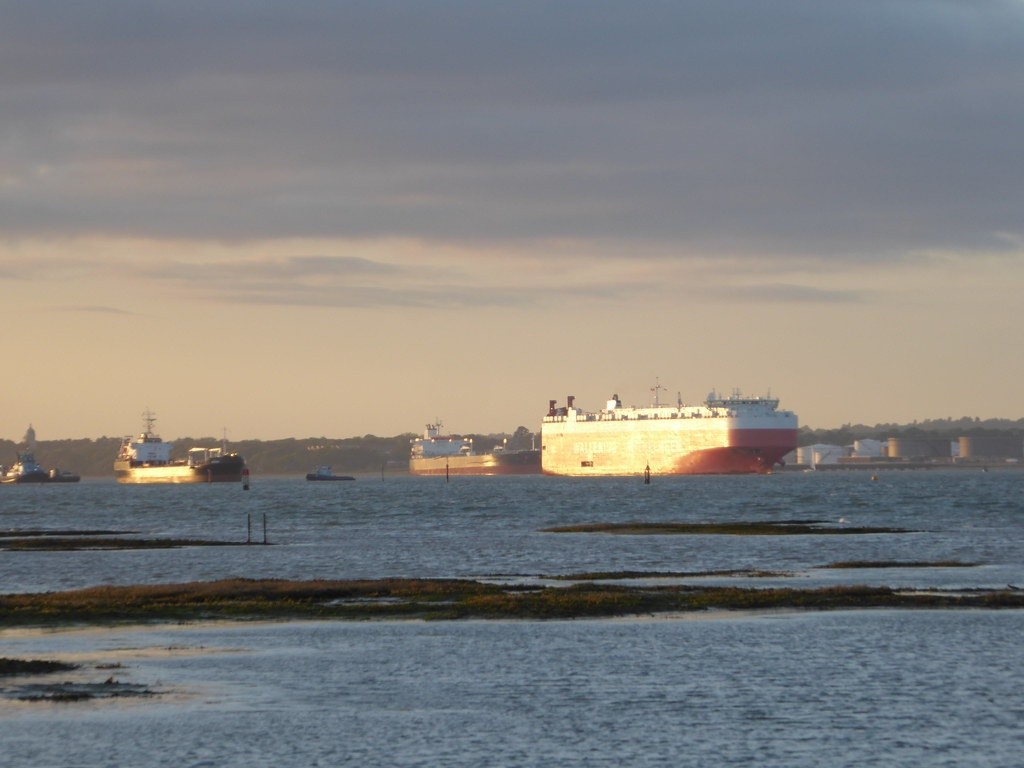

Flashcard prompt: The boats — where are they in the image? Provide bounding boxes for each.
[409,424,522,475]
[0,445,81,484]
[305,465,356,481]
[541,395,798,476]
[112,409,246,485]
